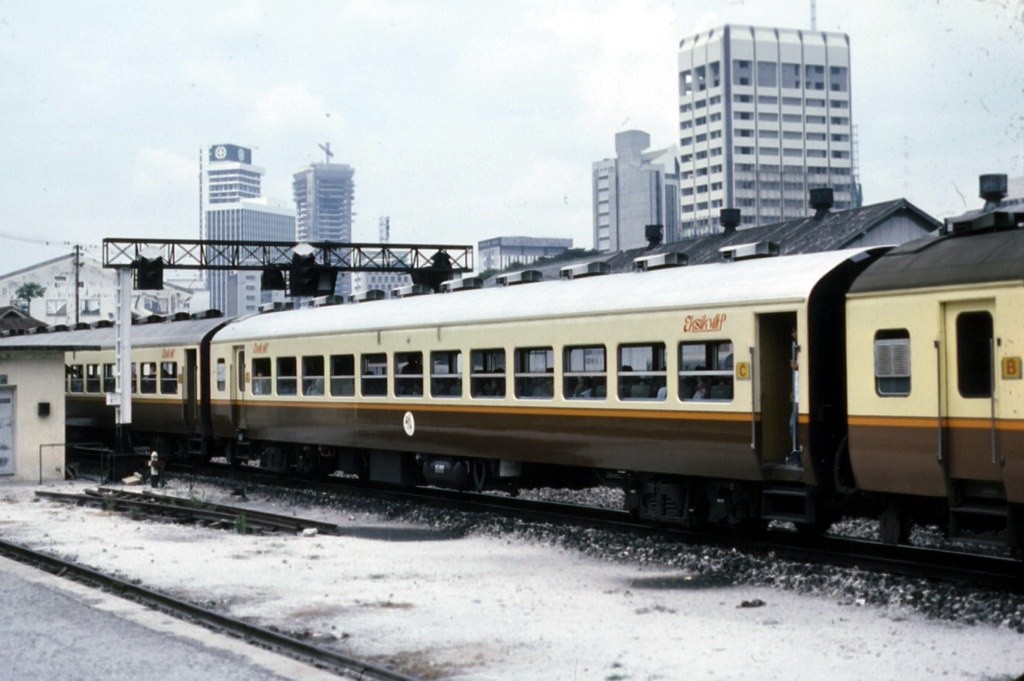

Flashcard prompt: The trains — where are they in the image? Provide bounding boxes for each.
[65,209,1024,544]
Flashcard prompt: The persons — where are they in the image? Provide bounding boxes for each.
[70,356,713,405]
